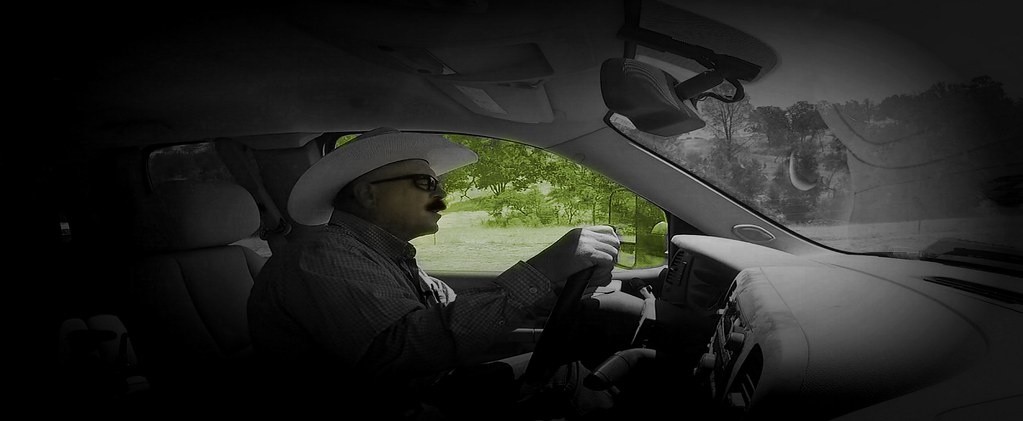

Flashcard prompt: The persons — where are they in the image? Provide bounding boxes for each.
[277,126,620,421]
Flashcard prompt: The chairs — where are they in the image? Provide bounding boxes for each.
[144,176,274,355]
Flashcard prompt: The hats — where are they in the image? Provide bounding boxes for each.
[287,126,478,226]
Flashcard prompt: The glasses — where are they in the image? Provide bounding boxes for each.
[365,173,441,192]
